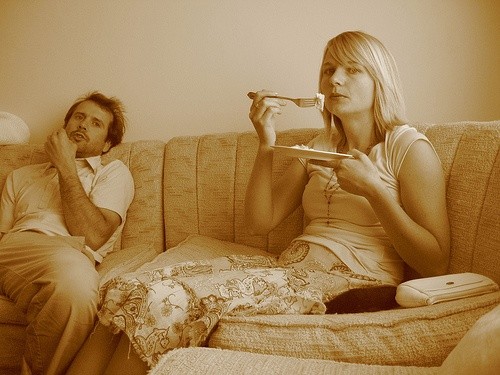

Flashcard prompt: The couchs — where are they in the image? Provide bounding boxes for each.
[0,121,500,375]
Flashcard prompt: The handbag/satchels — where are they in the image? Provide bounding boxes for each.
[395,272,498,308]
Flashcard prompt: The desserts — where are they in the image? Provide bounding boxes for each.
[315,92,325,112]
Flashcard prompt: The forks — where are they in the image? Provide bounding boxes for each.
[247,92,317,108]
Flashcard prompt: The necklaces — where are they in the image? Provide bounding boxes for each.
[323,143,374,223]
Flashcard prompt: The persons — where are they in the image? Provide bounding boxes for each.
[0,93,135,375]
[66,31,452,375]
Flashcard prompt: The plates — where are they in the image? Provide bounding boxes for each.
[270,146,355,161]
